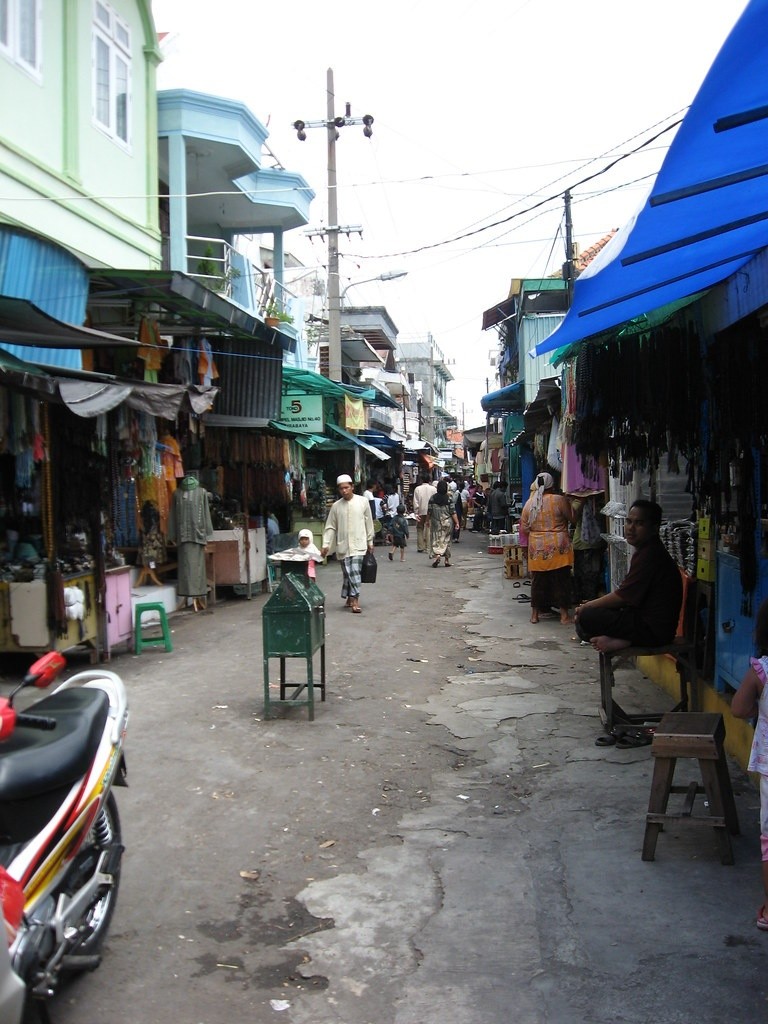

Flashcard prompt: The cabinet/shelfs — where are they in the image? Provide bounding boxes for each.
[0,565,134,665]
[713,551,768,728]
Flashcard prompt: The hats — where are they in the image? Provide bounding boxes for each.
[180,476,199,491]
[337,474,352,485]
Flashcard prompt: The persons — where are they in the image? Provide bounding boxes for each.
[425,480,460,568]
[322,474,375,613]
[519,472,602,625]
[362,478,400,545]
[414,471,514,553]
[298,529,330,585]
[574,500,684,654]
[731,597,768,931]
[387,505,410,563]
[168,477,213,598]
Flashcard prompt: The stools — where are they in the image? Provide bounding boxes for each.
[133,602,171,654]
[641,712,741,867]
[597,637,699,736]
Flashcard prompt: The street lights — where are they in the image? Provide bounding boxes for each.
[327,271,410,383]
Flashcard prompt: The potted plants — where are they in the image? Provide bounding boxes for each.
[261,293,280,327]
[277,309,305,338]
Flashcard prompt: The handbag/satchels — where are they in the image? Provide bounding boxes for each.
[361,552,378,583]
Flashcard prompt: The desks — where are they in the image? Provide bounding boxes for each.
[693,580,715,681]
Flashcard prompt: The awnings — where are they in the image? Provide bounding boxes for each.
[282,366,401,409]
[462,424,503,449]
[483,296,515,329]
[527,0,768,356]
[509,375,563,447]
[199,411,409,464]
[0,267,299,421]
[481,379,525,411]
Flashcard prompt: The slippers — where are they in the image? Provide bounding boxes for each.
[512,593,532,603]
[523,580,531,586]
[595,727,652,748]
[756,904,768,929]
[538,613,560,618]
[513,582,520,588]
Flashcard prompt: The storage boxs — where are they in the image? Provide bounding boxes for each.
[697,517,716,582]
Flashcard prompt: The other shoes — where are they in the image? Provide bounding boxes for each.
[346,600,353,607]
[352,605,361,612]
[432,556,441,568]
[445,562,451,567]
[388,552,393,561]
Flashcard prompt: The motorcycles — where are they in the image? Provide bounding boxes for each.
[0,640,129,1024]
